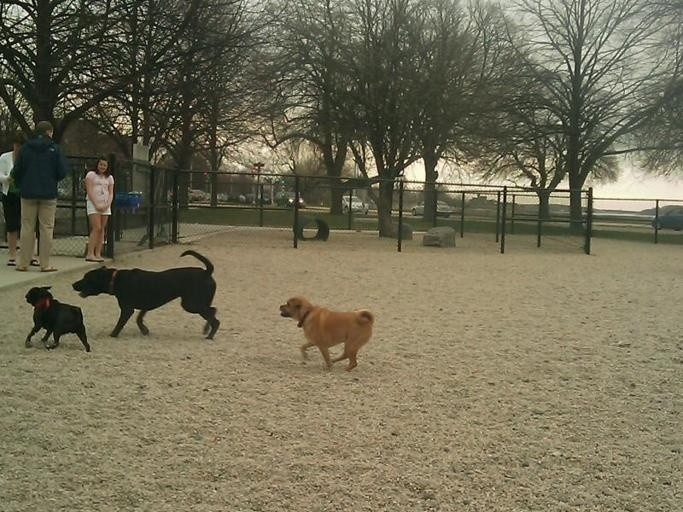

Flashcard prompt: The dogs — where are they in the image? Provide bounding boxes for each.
[72,250,220,339]
[280,297,374,372]
[26,287,89,352]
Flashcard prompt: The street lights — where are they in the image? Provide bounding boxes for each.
[254,162,265,203]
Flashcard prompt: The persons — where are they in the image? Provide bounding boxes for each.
[84,155,116,263]
[7,119,69,272]
[0,130,41,266]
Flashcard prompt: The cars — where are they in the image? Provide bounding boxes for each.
[343,196,368,213]
[188,188,303,208]
[652,208,683,230]
[410,200,455,218]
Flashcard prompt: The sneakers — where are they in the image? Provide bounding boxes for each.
[16,266,27,271]
[31,259,40,265]
[8,260,16,265]
[85,256,104,262]
[41,266,57,272]
